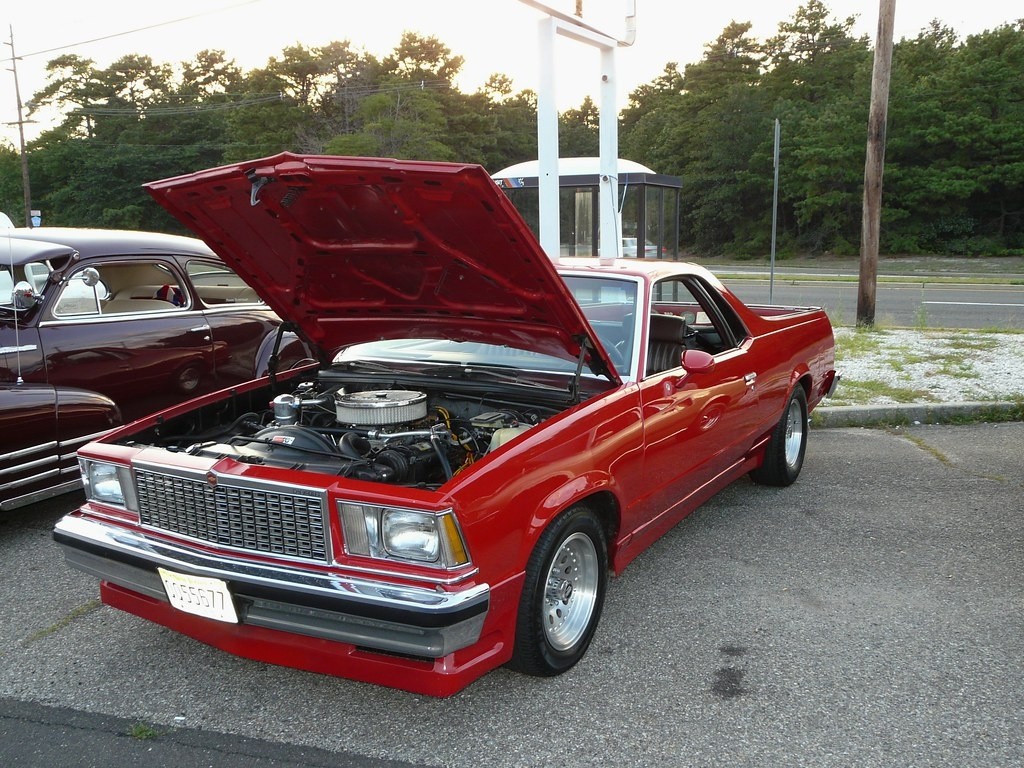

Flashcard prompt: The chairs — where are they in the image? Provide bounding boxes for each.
[622,311,687,374]
[56,297,108,313]
[101,300,179,312]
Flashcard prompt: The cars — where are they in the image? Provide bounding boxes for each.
[0,212,106,303]
[52,151,842,700]
[597,238,667,260]
[0,227,319,512]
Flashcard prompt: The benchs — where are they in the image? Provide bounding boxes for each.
[115,284,259,304]
